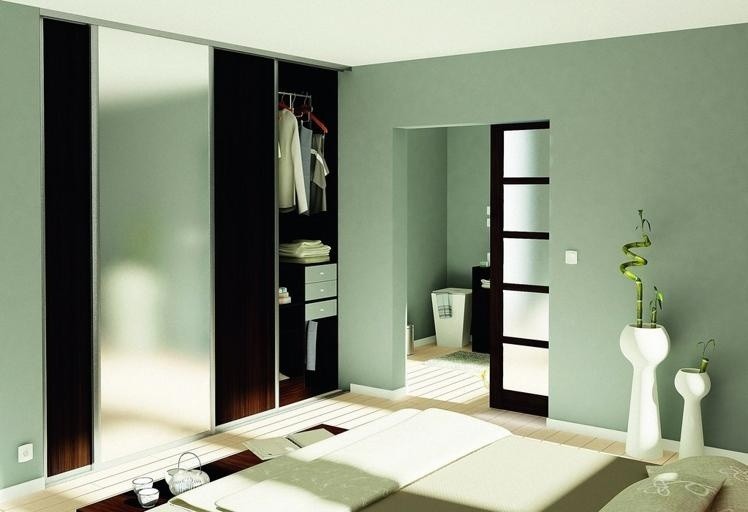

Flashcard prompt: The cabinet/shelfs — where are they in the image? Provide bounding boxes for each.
[0,0,355,496]
[471,265,490,356]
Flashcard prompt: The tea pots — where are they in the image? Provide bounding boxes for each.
[168,451,210,495]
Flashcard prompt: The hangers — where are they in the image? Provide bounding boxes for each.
[278,90,329,137]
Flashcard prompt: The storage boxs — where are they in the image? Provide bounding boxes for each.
[429,286,472,349]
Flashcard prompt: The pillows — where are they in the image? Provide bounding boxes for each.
[644,454,747,512]
[597,471,728,511]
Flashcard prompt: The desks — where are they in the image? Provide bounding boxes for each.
[73,423,351,512]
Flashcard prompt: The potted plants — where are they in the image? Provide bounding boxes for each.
[617,208,673,461]
[674,338,718,461]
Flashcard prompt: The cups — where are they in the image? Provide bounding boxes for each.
[132,467,186,508]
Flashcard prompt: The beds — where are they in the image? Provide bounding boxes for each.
[143,407,748,512]
